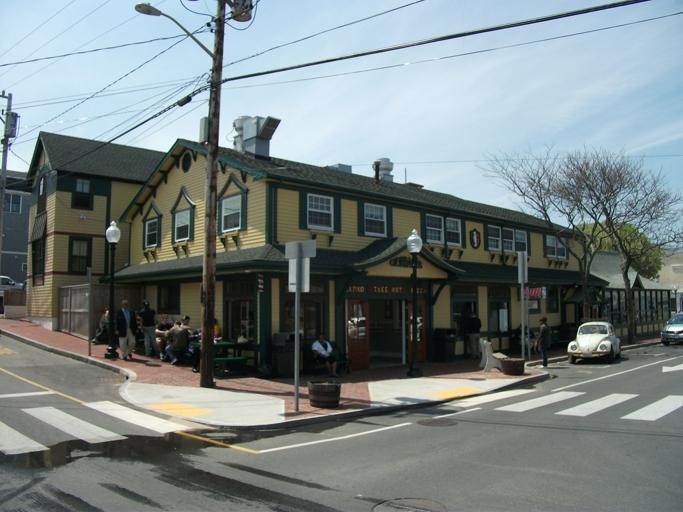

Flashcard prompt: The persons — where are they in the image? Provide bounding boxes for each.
[312,333,342,379]
[534,317,548,369]
[465,310,482,363]
[90,305,110,344]
[164,320,185,366]
[213,318,220,343]
[512,322,540,357]
[138,299,160,357]
[115,299,140,361]
[154,312,173,360]
[179,314,193,334]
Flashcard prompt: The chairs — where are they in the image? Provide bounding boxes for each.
[478,338,511,373]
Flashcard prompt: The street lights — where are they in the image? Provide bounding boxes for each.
[135,3,224,387]
[407,228,423,377]
[105,220,121,361]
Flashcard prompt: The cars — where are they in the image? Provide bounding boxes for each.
[0,276,24,296]
[661,311,683,346]
[567,321,621,364]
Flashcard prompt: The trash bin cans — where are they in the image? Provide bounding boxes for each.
[434,328,467,362]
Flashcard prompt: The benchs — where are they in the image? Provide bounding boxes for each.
[214,356,249,378]
[304,341,354,376]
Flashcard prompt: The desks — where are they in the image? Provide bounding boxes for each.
[189,339,236,377]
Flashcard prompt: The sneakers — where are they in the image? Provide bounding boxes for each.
[170,357,179,365]
[160,353,165,360]
[192,367,199,373]
[124,353,133,360]
